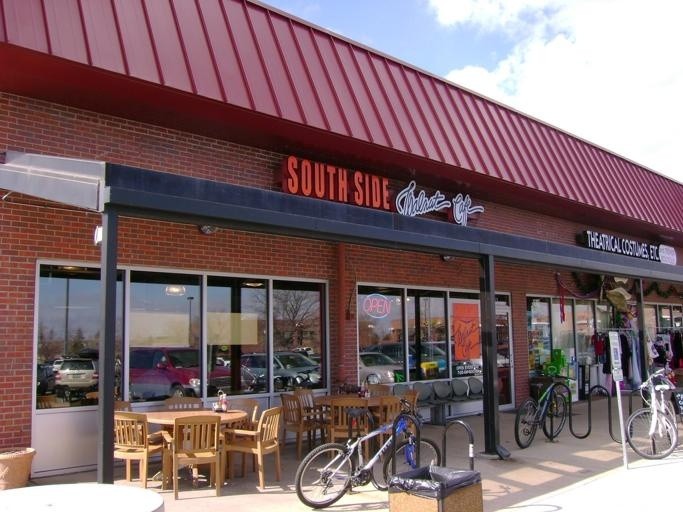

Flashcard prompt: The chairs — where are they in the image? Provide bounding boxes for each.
[393,377,484,426]
[113,397,285,500]
[280,383,420,469]
[36,391,99,409]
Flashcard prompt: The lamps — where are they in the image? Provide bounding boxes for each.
[164,285,186,297]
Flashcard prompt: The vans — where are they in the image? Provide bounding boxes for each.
[227,352,321,387]
[532,315,679,331]
[122,348,243,398]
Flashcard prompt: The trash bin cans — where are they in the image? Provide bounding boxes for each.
[388,466,483,512]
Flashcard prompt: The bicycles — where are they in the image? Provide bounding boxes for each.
[514,373,576,449]
[295,398,440,508]
[625,367,679,460]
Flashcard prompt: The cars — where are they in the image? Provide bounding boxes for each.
[54,358,98,396]
[298,347,313,352]
[359,339,508,385]
[53,360,63,372]
[37,367,55,394]
